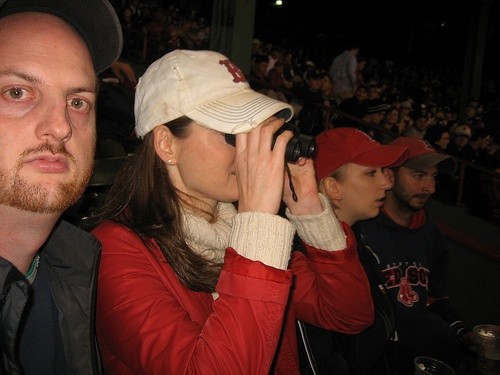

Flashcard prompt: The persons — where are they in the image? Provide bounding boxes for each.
[0,11,103,375]
[88,50,374,375]
[351,136,500,375]
[83,36,500,238]
[296,129,412,375]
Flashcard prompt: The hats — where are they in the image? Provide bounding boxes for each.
[417,103,427,110]
[400,102,413,111]
[133,49,294,139]
[454,125,471,138]
[388,137,457,172]
[306,69,324,81]
[312,127,408,181]
[0,0,124,73]
[365,98,386,113]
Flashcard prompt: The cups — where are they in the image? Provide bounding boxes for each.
[414,356,454,375]
[473,324,500,375]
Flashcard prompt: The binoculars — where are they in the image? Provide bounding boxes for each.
[224,119,316,162]
